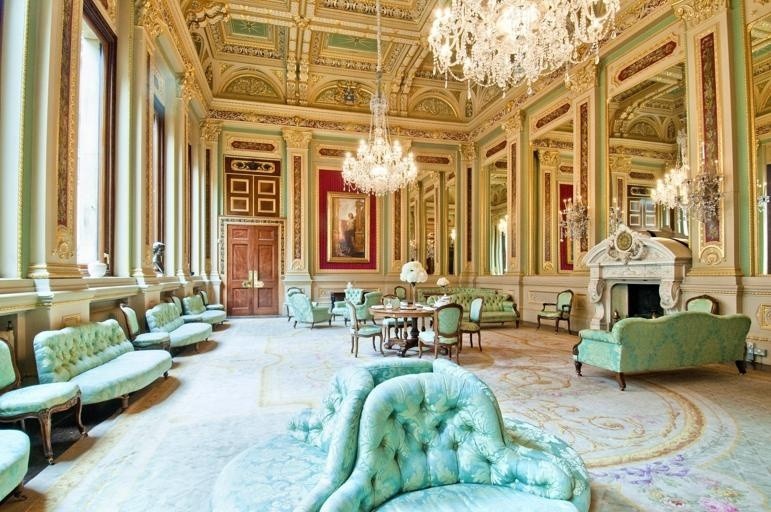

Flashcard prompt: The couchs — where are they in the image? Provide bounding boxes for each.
[183,294,226,331]
[0,430,31,502]
[33,318,172,409]
[144,302,212,353]
[572,314,752,391]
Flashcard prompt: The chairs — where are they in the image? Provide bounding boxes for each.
[200,291,223,325]
[167,295,202,323]
[120,303,171,352]
[685,295,720,315]
[0,337,85,465]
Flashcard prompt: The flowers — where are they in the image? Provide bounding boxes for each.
[401,262,428,283]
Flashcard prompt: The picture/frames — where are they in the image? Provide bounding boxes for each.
[327,192,370,263]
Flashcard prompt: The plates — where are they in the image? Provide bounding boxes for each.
[371,301,430,310]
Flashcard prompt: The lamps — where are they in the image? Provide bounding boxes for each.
[688,164,725,218]
[757,179,770,213]
[428,0,621,99]
[341,0,417,196]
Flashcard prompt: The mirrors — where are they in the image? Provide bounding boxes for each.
[740,0,771,276]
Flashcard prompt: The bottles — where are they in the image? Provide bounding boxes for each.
[88,260,109,278]
[346,281,354,288]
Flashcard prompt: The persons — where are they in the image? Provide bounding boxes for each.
[151,241,165,273]
[339,212,360,259]
